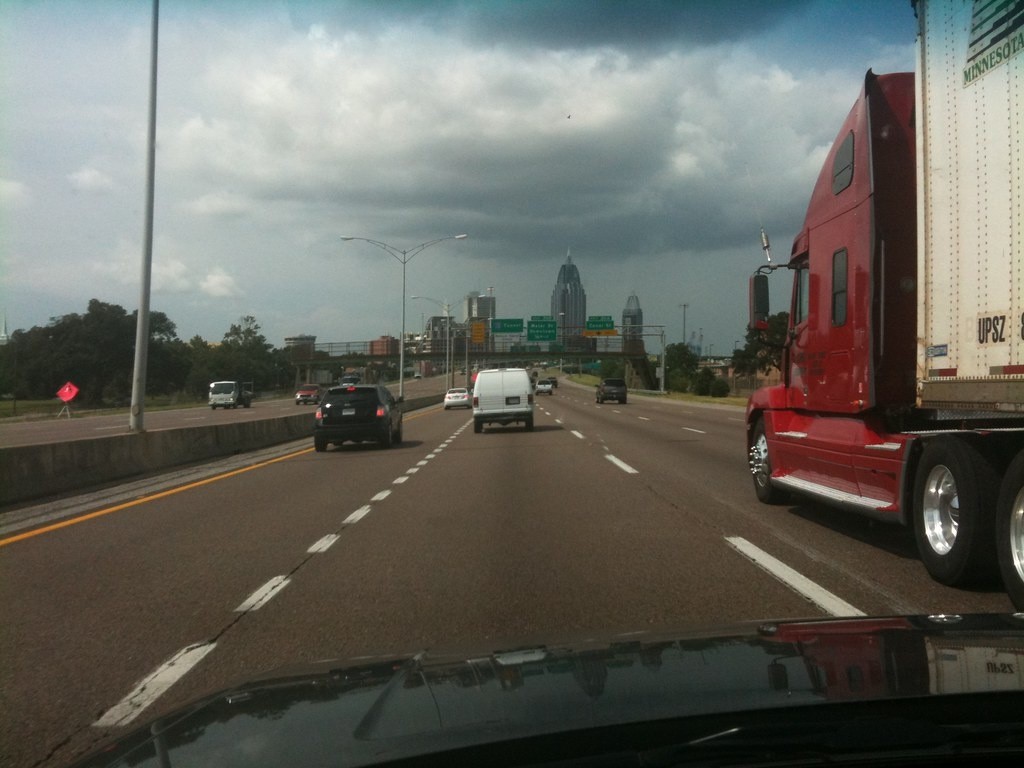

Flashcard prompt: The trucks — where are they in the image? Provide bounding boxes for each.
[208,380,254,409]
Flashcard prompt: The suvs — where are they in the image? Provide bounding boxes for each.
[594,378,628,405]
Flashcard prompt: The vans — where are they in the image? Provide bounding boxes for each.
[338,374,362,389]
[467,367,537,433]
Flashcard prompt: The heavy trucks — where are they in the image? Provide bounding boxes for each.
[744,0,1024,627]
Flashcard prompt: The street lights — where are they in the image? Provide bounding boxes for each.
[340,233,469,403]
[410,294,490,393]
[558,312,566,373]
[440,316,493,389]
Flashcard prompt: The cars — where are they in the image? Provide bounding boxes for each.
[531,359,559,371]
[547,376,559,388]
[529,370,538,387]
[294,383,326,406]
[443,387,473,410]
[534,378,553,396]
[414,372,423,380]
[314,383,406,450]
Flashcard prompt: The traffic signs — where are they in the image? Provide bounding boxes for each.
[527,319,557,341]
